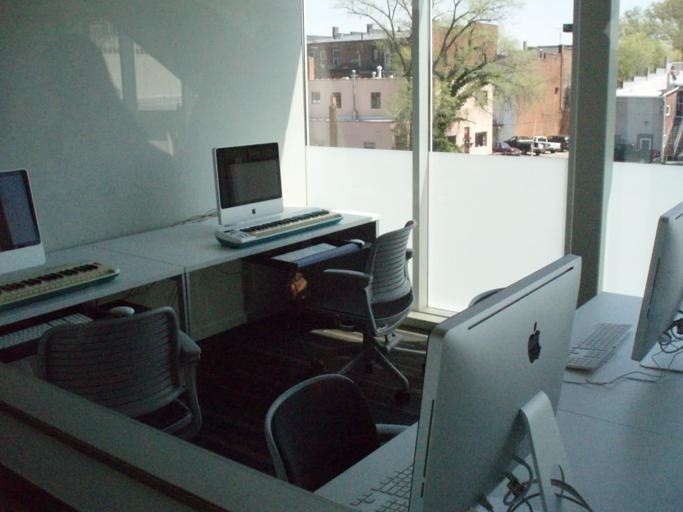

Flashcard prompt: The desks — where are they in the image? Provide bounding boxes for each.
[310,293,682,512]
[0,243,188,372]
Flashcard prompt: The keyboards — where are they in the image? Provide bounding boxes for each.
[565,321,633,374]
[0,311,94,351]
[270,242,337,264]
[341,456,415,512]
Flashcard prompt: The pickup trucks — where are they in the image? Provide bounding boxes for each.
[491,132,569,156]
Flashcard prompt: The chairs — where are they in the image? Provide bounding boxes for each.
[299,219,419,400]
[91,206,379,340]
[267,373,412,492]
[37,305,203,440]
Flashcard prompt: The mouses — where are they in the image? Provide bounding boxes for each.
[345,238,365,247]
[110,306,136,317]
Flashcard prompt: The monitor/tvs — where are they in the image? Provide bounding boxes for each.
[408,252,597,512]
[0,168,47,281]
[213,141,283,226]
[630,202,683,373]
